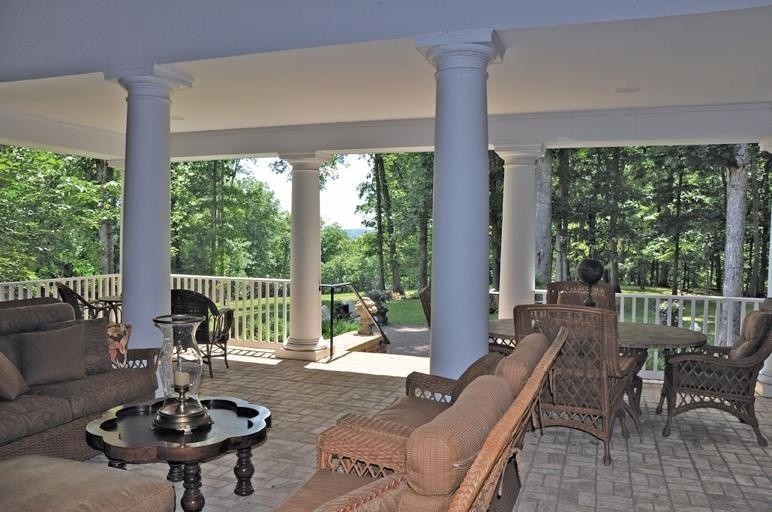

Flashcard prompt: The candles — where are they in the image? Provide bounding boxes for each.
[173,369,189,387]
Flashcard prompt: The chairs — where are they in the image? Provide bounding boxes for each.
[546,280,648,410]
[59,279,125,324]
[272,373,543,511]
[511,302,646,465]
[651,292,772,452]
[335,319,569,510]
[167,287,235,381]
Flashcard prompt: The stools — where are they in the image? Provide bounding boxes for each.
[0,451,175,510]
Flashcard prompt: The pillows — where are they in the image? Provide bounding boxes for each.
[1,316,134,399]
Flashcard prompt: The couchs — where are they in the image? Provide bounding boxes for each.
[2,296,163,462]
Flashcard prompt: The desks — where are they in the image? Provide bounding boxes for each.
[99,294,124,306]
[479,321,712,437]
